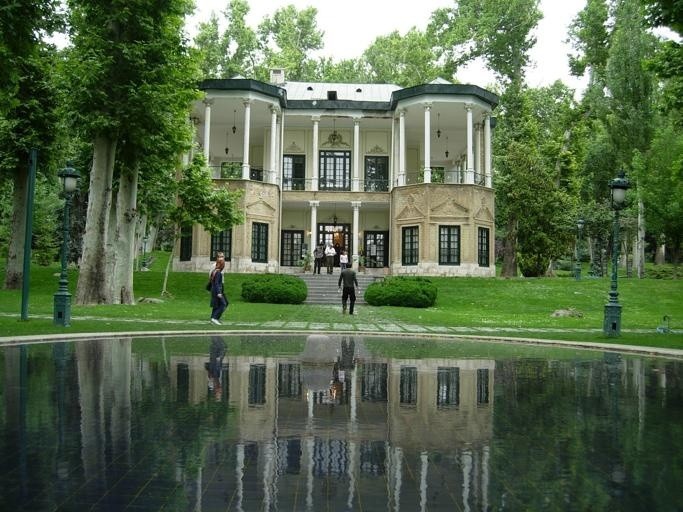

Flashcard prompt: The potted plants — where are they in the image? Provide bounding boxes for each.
[354,254,368,274]
[297,249,311,272]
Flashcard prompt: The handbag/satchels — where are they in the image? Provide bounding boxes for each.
[206,280,212,291]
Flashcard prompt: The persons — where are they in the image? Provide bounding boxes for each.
[338,262,361,316]
[207,251,229,327]
[327,336,356,407]
[203,334,228,405]
[313,237,348,276]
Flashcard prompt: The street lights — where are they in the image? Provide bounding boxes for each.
[53,160,84,328]
[603,168,632,341]
[139,236,148,273]
[575,219,584,279]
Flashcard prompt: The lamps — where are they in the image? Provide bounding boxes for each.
[436,112,442,139]
[445,136,449,158]
[232,109,237,134]
[224,132,229,155]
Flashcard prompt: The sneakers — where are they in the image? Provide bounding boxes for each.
[208,317,222,326]
